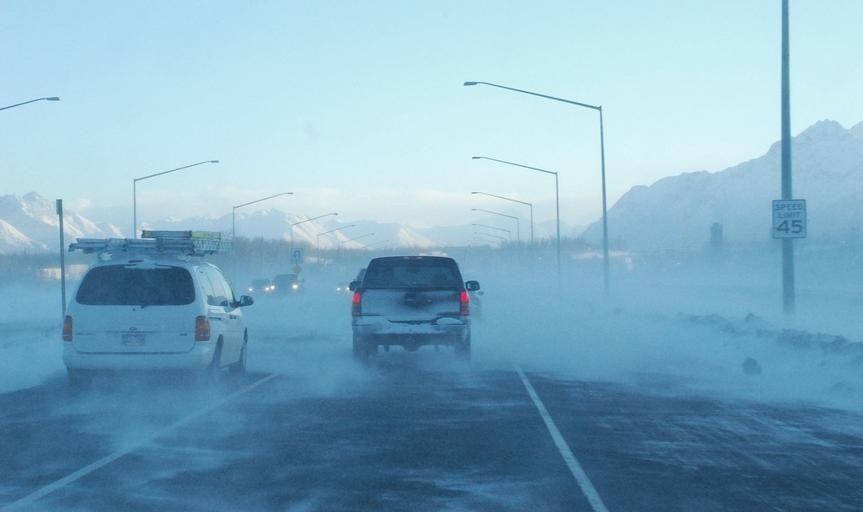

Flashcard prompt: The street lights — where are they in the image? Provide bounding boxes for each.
[232,191,294,237]
[341,232,375,252]
[316,224,356,263]
[463,81,612,306]
[0,97,60,111]
[133,159,220,236]
[290,213,338,246]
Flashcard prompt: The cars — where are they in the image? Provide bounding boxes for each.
[245,273,302,297]
[335,279,350,293]
[61,230,255,390]
[349,255,485,363]
